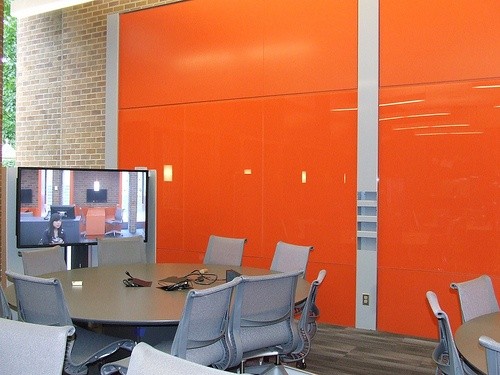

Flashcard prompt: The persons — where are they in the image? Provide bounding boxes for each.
[40,213,67,244]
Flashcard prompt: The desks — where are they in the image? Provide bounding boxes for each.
[454,311,500,375]
[86,208,105,239]
[6,262,314,345]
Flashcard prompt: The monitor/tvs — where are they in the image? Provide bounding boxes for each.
[50,205,76,219]
[16,166,149,244]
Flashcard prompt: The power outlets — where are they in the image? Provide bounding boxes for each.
[363,294,369,305]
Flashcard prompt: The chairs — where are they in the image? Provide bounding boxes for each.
[450,276,499,325]
[105,207,125,238]
[425,290,477,375]
[0,234,327,375]
[478,336,500,375]
[78,205,86,238]
